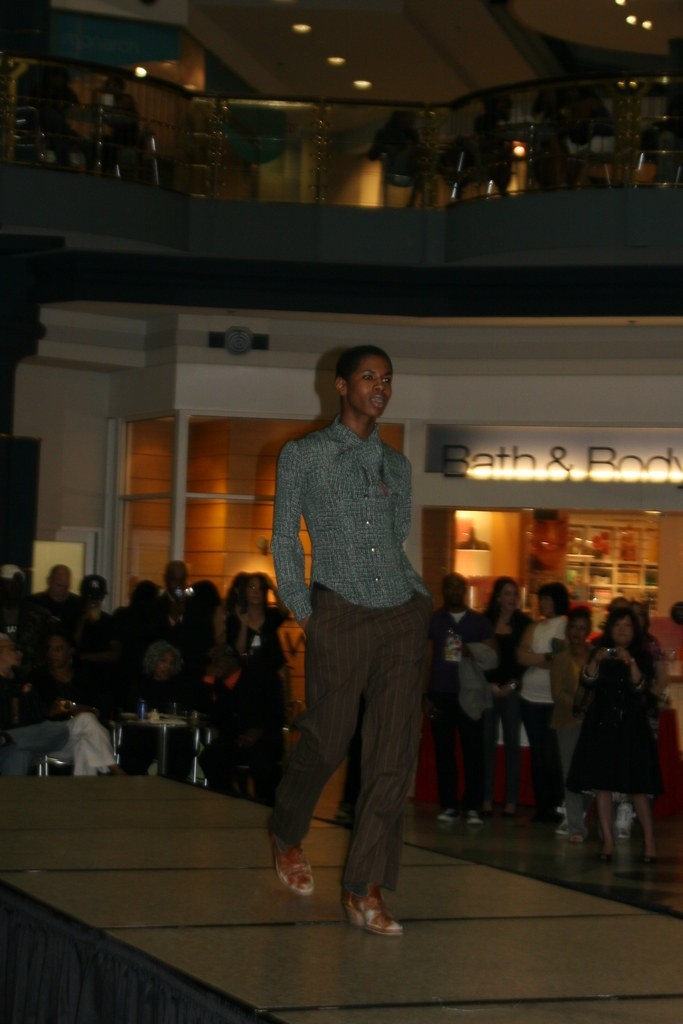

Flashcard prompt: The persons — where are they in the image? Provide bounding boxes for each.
[267,346,434,938]
[38,67,140,171]
[371,87,627,206]
[0,563,682,865]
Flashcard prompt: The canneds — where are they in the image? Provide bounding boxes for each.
[137,700,148,720]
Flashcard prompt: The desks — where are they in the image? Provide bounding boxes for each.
[487,122,555,188]
[121,712,208,775]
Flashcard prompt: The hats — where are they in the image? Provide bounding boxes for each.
[52,565,72,585]
[81,574,107,598]
[1,564,26,583]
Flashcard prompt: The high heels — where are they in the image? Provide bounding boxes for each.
[269,817,314,896]
[341,883,405,936]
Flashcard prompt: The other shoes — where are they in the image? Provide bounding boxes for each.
[436,808,460,822]
[600,845,616,861]
[570,833,584,842]
[642,841,657,863]
[555,818,569,835]
[466,809,483,824]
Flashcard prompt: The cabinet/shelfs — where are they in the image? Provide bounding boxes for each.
[566,519,660,605]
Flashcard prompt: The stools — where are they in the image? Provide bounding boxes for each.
[234,763,252,798]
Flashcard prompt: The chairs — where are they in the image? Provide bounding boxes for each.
[380,120,683,204]
[12,664,123,777]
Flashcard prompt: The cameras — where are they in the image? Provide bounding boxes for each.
[601,646,618,659]
[59,699,76,711]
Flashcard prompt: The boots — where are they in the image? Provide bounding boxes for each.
[615,803,636,838]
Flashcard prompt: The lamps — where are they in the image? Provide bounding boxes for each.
[456,522,490,552]
